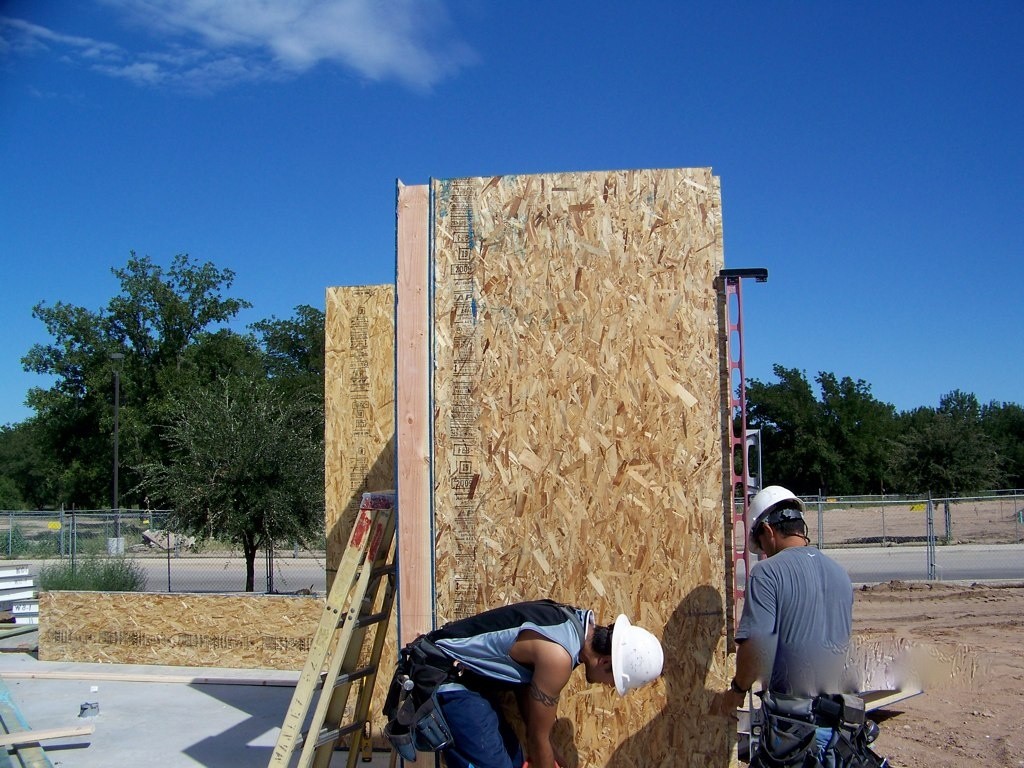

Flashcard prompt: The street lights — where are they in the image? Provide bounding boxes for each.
[110,353,126,538]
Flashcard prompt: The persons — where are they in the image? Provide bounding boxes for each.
[408,599,664,768]
[713,485,891,768]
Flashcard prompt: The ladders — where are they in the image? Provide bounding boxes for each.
[267,490,398,768]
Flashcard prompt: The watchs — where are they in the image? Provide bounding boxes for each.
[731,677,752,695]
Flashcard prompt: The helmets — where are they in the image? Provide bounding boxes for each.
[747,486,806,554]
[611,614,664,696]
